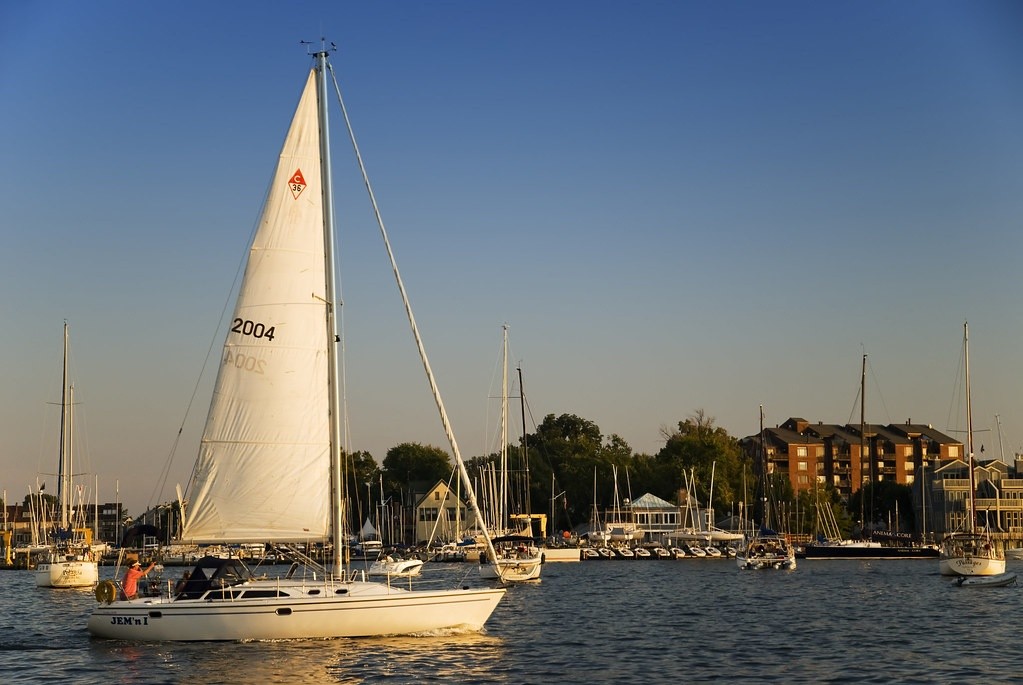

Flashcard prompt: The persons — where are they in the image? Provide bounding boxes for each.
[120,560,154,600]
[175,570,191,596]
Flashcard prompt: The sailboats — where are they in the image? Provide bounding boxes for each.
[736,402,797,571]
[478,316,544,583]
[30,317,100,588]
[580,463,740,559]
[938,320,1007,577]
[87,33,510,644]
[797,349,945,559]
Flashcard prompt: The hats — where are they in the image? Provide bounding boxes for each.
[132,560,141,567]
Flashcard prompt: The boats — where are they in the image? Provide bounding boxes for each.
[366,544,425,580]
[158,537,333,564]
[953,571,1018,592]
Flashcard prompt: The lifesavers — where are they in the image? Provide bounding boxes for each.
[96,580,116,602]
